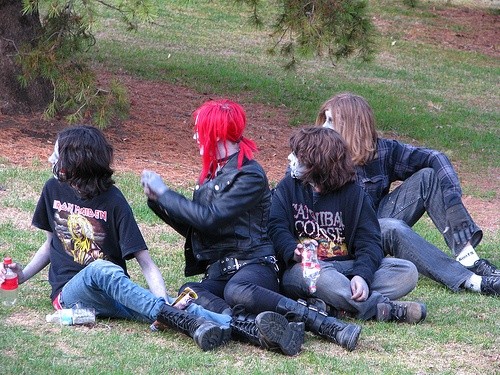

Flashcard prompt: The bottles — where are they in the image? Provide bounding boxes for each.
[1,257,19,306]
[45,307,96,325]
[301,239,320,293]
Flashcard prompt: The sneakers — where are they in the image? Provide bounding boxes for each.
[306,297,339,319]
[377,301,427,324]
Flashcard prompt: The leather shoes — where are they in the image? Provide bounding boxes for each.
[466,258,500,277]
[481,274,499,296]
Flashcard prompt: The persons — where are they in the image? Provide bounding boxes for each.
[316,93,500,297]
[0,126,301,355]
[269,126,427,324]
[142,99,362,352]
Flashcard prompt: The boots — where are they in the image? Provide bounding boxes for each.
[229,303,305,357]
[156,302,232,351]
[275,297,363,351]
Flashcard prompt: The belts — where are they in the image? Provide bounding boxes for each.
[205,247,272,277]
